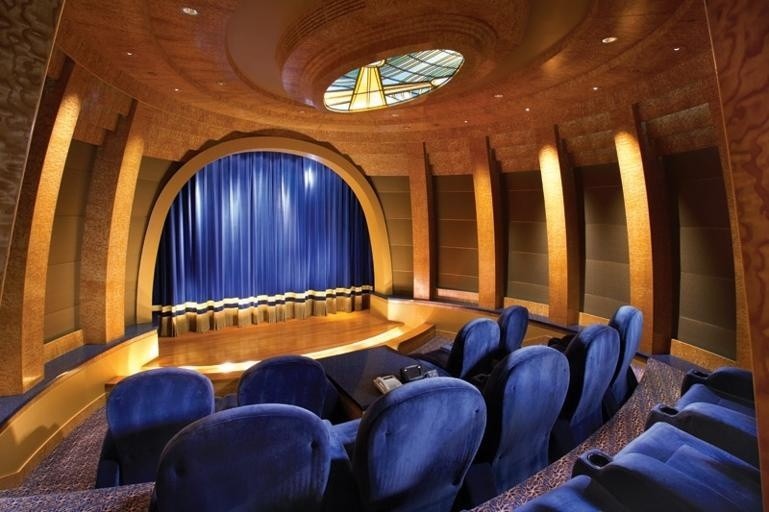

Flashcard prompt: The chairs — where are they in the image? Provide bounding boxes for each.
[497,305,528,354]
[219,355,333,417]
[150,403,330,512]
[602,305,642,418]
[512,475,628,512]
[95,368,215,488]
[570,427,760,512]
[455,346,570,504]
[416,318,500,378]
[549,325,620,454]
[645,368,758,466]
[320,377,487,512]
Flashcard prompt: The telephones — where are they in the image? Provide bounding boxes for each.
[373,374,408,394]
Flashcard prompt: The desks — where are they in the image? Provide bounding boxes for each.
[314,345,451,417]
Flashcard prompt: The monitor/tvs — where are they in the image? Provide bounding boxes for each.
[400,364,425,382]
